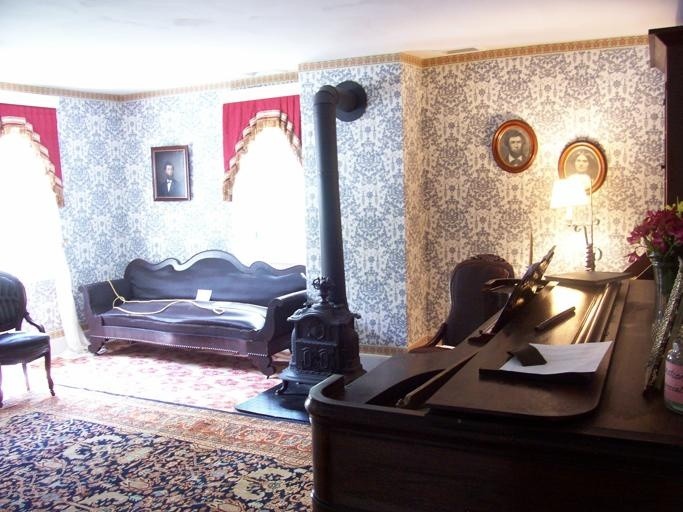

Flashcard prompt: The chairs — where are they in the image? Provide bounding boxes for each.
[0,271,55,406]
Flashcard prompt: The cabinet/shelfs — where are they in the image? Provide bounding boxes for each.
[620,24,682,280]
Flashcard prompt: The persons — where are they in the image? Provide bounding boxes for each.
[503,129,527,167]
[571,152,596,175]
[159,163,183,196]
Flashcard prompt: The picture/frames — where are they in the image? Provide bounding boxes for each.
[557,141,608,196]
[491,119,538,174]
[149,145,191,202]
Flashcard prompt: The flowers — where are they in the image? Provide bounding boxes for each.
[622,197,683,303]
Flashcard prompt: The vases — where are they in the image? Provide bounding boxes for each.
[646,251,682,357]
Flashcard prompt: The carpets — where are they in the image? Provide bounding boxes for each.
[0,380,312,511]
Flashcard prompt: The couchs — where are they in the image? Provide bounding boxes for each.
[75,250,306,377]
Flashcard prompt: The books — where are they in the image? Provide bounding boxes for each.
[545,270,634,286]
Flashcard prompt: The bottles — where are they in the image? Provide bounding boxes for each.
[662,338,683,415]
